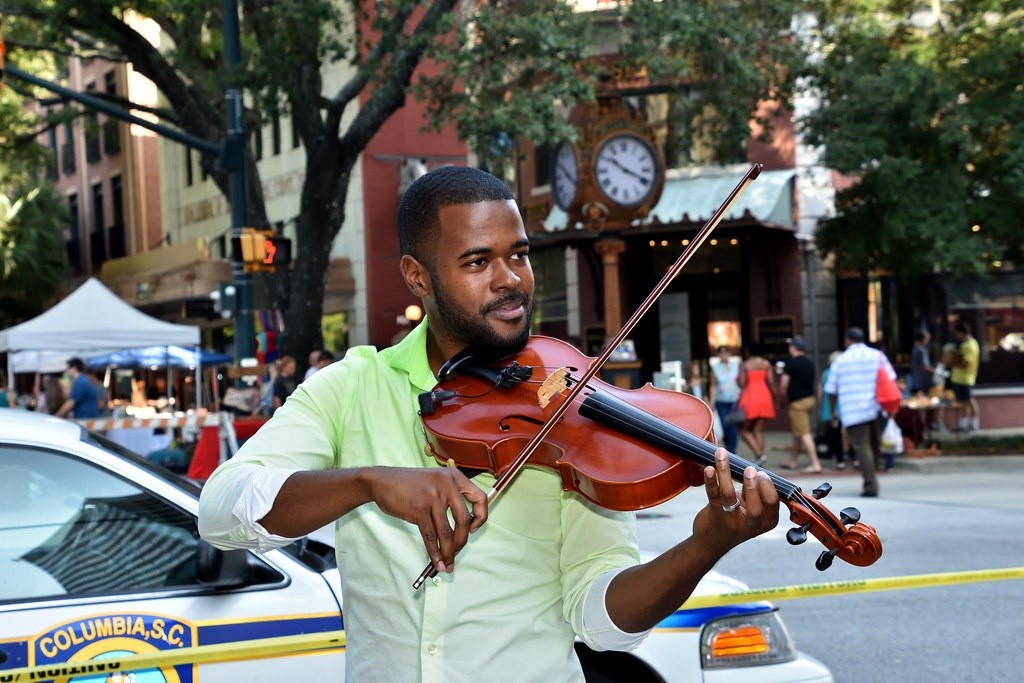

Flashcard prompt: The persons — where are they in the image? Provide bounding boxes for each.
[197,165,780,682]
[39,348,335,419]
[709,315,980,497]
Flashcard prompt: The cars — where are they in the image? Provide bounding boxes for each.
[0,404,837,683]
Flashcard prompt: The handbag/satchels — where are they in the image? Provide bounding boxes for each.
[725,407,747,428]
[815,422,833,460]
[876,354,902,412]
[879,418,905,454]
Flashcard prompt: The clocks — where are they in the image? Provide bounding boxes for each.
[554,139,578,210]
[595,134,659,206]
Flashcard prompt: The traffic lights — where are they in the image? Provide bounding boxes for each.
[231,232,256,263]
[263,236,293,267]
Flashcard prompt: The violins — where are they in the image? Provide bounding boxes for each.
[419,333,885,569]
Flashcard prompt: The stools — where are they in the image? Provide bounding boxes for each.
[937,399,964,431]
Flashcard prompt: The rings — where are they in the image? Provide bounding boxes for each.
[722,498,741,512]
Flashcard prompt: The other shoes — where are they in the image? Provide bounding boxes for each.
[861,491,877,496]
[754,454,767,466]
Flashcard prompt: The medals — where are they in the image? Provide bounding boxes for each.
[0,275,202,414]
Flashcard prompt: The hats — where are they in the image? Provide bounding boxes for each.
[787,335,806,350]
